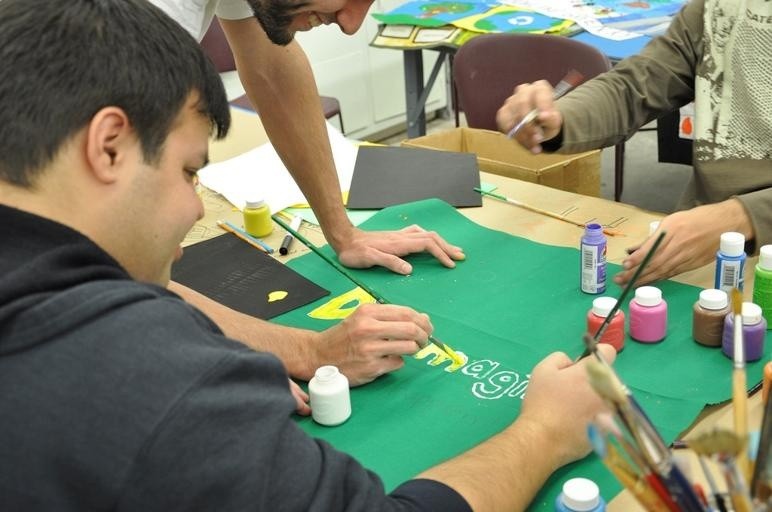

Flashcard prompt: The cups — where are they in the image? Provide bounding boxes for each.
[554,477,608,511]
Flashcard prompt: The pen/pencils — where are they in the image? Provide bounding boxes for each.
[280,217,303,256]
[218,218,274,254]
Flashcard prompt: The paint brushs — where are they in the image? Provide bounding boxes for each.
[270,213,463,368]
[580,231,666,360]
[505,68,584,142]
[587,287,772,511]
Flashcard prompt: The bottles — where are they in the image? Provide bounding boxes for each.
[752,243,772,330]
[308,365,352,428]
[579,224,607,294]
[720,303,766,364]
[714,230,748,298]
[692,287,731,346]
[243,193,273,238]
[629,286,666,342]
[586,296,625,353]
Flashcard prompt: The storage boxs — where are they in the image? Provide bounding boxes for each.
[394,123,604,201]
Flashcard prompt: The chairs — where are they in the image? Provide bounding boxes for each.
[453,32,625,202]
[202,11,346,132]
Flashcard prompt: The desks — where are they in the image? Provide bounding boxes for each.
[378,23,626,139]
[157,104,772,512]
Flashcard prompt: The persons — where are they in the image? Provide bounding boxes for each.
[150,0,464,388]
[1,0,615,510]
[497,0,772,289]
